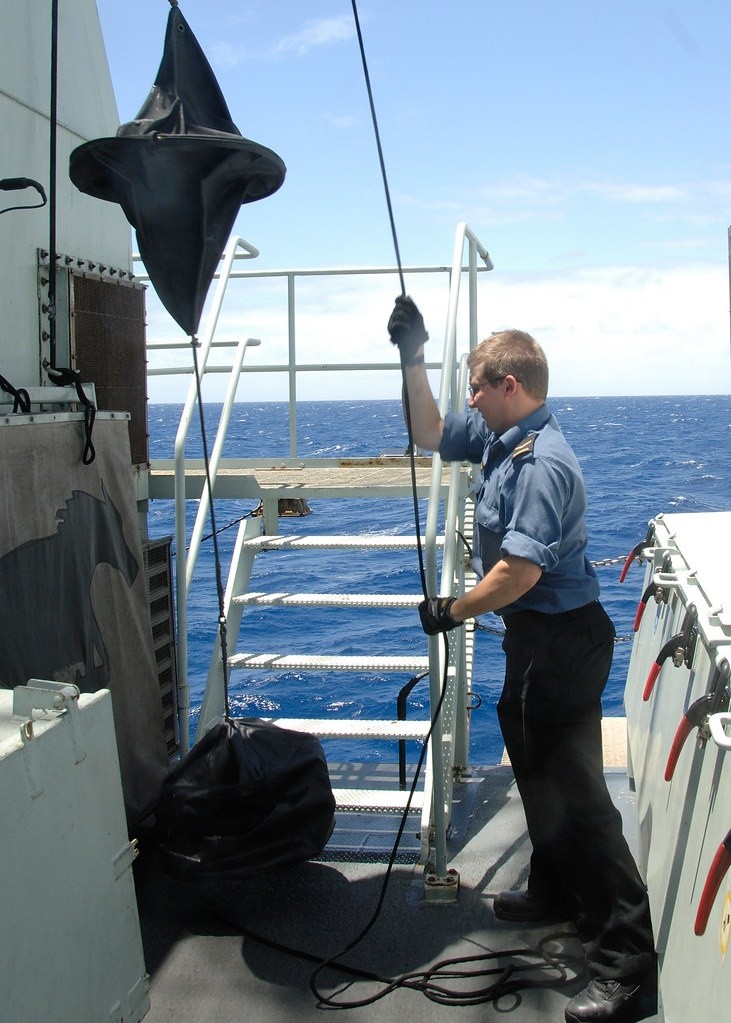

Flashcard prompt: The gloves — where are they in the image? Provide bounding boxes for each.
[418,598,464,636]
[388,296,429,348]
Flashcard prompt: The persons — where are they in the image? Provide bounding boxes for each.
[388,295,658,1023]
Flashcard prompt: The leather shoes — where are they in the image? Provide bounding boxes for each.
[493,888,560,921]
[565,974,642,1023]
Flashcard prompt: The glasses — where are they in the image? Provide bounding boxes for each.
[468,376,521,398]
